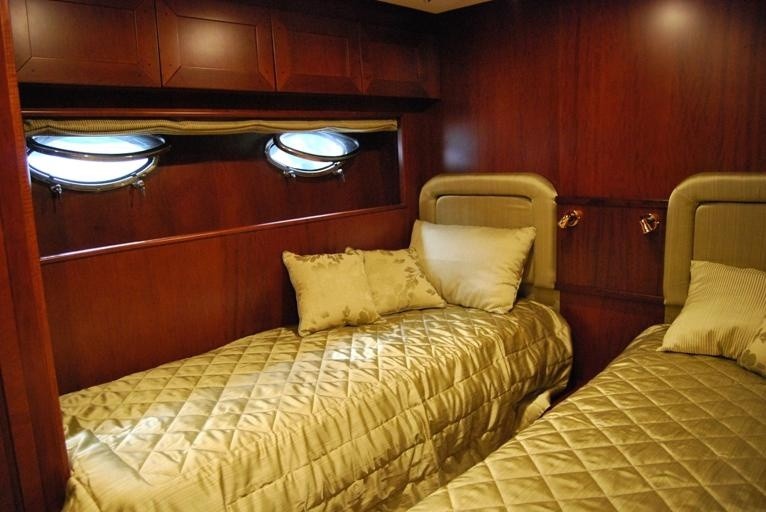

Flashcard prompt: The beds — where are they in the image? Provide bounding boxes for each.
[409,167,765,512]
[58,171,575,512]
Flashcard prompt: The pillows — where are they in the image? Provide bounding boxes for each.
[658,258,766,360]
[408,219,537,315]
[345,243,448,319]
[738,316,766,377]
[282,249,390,337]
